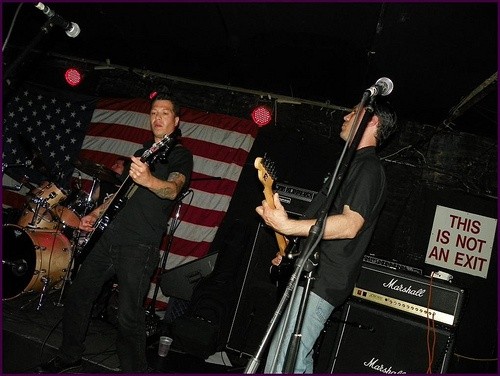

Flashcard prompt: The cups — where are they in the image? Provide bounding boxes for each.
[158,336,173,357]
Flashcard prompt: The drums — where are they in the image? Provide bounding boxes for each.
[1,222,73,302]
[31,179,68,207]
[53,205,82,239]
[17,201,60,229]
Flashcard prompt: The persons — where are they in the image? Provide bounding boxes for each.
[34,92,192,374]
[256,96,397,374]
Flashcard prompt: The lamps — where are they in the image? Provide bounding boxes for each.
[64,63,95,86]
[252,98,276,126]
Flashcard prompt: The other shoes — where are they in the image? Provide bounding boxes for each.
[38,353,84,373]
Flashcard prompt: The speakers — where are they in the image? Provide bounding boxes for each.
[228,215,295,360]
[313,298,457,374]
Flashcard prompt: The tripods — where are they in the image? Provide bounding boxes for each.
[19,174,99,311]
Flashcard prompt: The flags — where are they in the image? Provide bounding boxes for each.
[2,72,259,312]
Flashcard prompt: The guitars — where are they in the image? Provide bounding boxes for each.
[253,150,302,307]
[73,129,181,259]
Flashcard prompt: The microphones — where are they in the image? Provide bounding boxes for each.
[177,190,192,203]
[364,76,394,98]
[34,2,81,38]
[354,322,375,332]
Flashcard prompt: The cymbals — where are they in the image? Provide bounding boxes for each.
[5,169,35,189]
[74,159,124,185]
